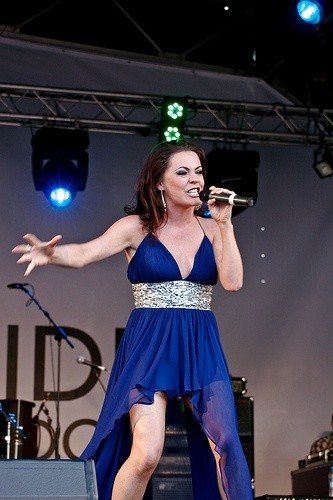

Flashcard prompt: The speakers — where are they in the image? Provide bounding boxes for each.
[141,395,255,500]
[291,461,333,500]
[0,458,98,500]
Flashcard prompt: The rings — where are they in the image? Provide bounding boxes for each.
[26,244,31,252]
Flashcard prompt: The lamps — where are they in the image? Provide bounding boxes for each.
[311,141,333,179]
[203,147,261,217]
[31,125,90,207]
[158,96,197,145]
[296,0,328,25]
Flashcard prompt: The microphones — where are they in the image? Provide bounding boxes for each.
[7,282,29,288]
[199,190,253,207]
[76,356,106,371]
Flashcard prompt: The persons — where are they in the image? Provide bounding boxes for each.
[12,142,252,500]
